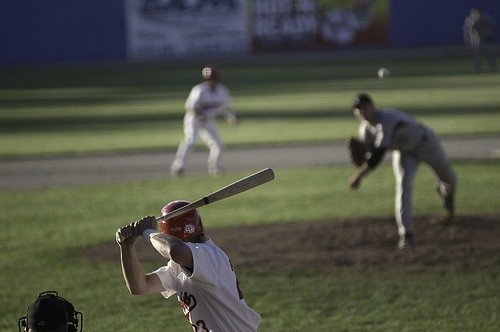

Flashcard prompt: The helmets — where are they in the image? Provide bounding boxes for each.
[159,200,205,242]
[26,294,74,332]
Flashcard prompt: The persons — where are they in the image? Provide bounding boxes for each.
[344,92,458,250]
[166,63,237,184]
[16,288,84,332]
[116,201,263,332]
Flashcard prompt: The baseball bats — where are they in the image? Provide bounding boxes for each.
[155,169,278,223]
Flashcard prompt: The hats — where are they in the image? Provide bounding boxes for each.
[351,93,371,109]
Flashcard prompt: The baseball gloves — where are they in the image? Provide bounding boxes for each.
[348,136,367,166]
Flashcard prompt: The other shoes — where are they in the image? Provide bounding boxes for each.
[398,231,417,250]
